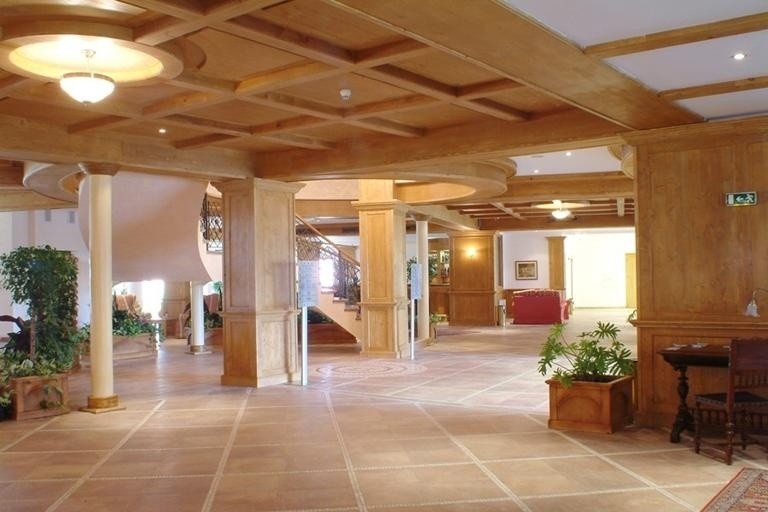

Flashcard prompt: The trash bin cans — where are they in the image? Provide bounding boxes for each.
[498,305,505,326]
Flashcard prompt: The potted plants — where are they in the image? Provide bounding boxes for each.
[81,310,163,362]
[0,245,79,421]
[536,320,635,434]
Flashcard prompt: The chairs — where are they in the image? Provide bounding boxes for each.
[694,336,768,465]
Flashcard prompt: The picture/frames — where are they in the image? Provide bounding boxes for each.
[515,261,538,280]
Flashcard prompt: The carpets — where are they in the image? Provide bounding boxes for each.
[698,466,768,512]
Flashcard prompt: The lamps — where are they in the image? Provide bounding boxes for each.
[58,48,116,108]
[551,207,571,220]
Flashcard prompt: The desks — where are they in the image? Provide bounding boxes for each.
[656,344,730,443]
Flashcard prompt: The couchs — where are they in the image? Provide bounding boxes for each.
[513,289,567,325]
